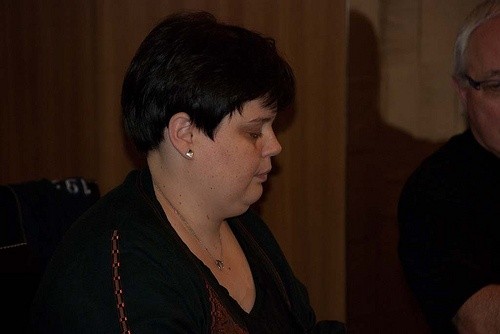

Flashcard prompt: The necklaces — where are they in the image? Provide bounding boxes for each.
[153,181,224,273]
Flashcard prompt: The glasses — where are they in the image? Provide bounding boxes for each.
[460,71,500,94]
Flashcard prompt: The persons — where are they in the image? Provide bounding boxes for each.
[25,12,319,334]
[397,0,500,334]
